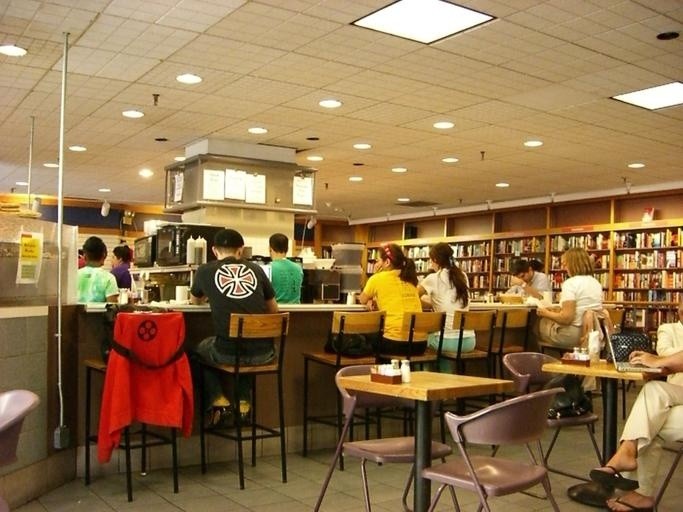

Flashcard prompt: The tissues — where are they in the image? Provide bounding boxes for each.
[562,352,590,366]
[371,366,400,383]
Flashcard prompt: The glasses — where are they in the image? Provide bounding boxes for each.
[520,273,527,278]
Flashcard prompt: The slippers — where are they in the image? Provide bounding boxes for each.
[607,497,654,512]
[589,465,639,491]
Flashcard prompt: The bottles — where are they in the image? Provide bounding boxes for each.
[195,236,203,264]
[573,347,579,353]
[202,237,207,264]
[580,348,587,353]
[401,360,411,383]
[119,288,129,304]
[391,359,399,369]
[186,235,196,264]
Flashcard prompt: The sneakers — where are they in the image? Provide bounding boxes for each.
[207,396,234,430]
[240,401,250,428]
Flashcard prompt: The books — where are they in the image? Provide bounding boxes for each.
[495,291,504,302]
[494,256,518,271]
[614,228,683,250]
[589,252,611,268]
[473,274,490,289]
[613,289,683,303]
[614,271,683,288]
[366,248,380,274]
[448,242,491,257]
[602,289,609,301]
[642,207,655,221]
[521,256,544,265]
[495,235,546,252]
[550,233,609,250]
[492,273,523,287]
[548,272,570,288]
[406,246,435,283]
[552,291,562,303]
[551,254,565,269]
[470,290,490,300]
[591,272,608,286]
[622,309,680,332]
[451,258,490,272]
[615,250,683,270]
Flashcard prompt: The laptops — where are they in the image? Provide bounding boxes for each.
[596,317,663,373]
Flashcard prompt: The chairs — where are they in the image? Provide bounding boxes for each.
[83,312,181,502]
[421,387,565,512]
[441,308,497,450]
[365,311,446,466]
[200,312,290,490]
[653,440,682,512]
[314,364,461,512]
[0,388,41,511]
[302,310,386,470]
[537,307,609,353]
[479,307,530,407]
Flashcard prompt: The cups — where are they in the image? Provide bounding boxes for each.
[542,291,551,308]
[176,285,188,302]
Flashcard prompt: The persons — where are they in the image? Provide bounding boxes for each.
[190,229,277,427]
[590,349,683,512]
[532,248,603,348]
[75,236,120,303]
[108,246,132,289]
[416,242,476,353]
[657,300,683,385]
[360,243,429,356]
[263,233,303,303]
[499,260,553,305]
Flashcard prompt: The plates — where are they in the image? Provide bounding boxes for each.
[313,258,336,269]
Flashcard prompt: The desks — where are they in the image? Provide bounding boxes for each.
[541,363,668,508]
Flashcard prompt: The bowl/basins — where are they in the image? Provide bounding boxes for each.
[499,295,522,306]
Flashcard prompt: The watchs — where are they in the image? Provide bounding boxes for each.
[521,281,527,291]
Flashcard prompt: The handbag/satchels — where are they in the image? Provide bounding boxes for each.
[324,329,377,359]
[606,307,651,363]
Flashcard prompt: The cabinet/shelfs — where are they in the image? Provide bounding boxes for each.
[292,181,682,353]
